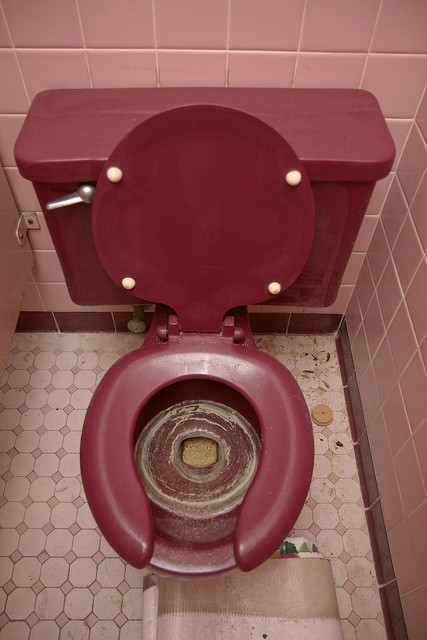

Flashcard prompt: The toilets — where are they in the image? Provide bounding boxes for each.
[12,87,396,579]
